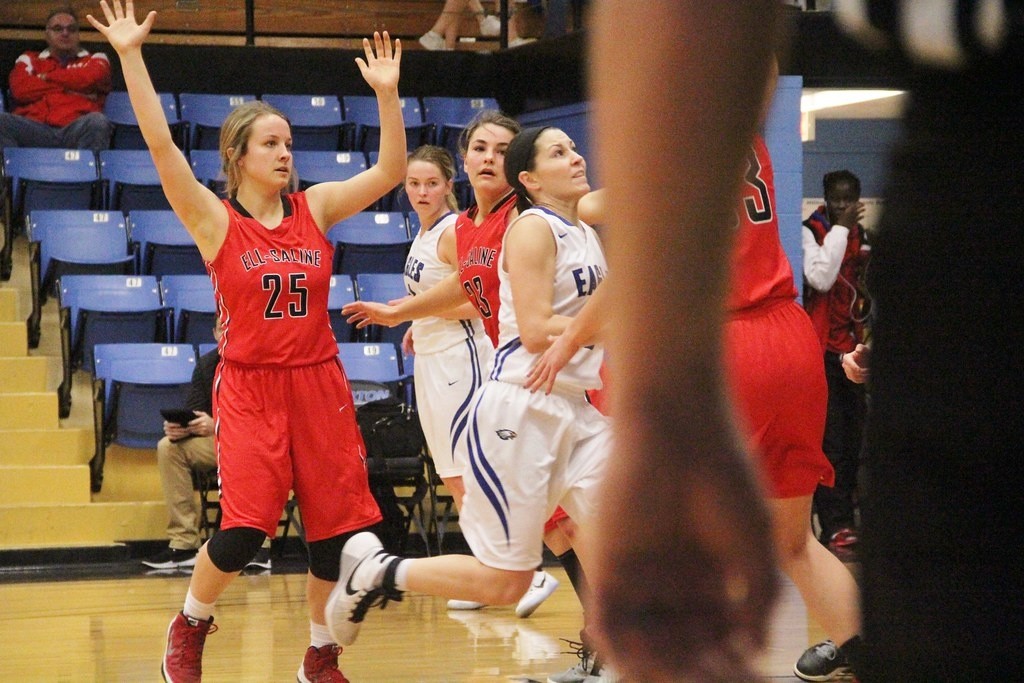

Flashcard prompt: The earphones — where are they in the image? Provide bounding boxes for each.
[824,200,827,206]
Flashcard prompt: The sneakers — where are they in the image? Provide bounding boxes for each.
[323,532,397,649]
[296,643,350,683]
[446,597,488,612]
[160,609,219,683]
[515,570,560,618]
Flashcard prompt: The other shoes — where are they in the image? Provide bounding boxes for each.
[419,31,442,52]
[828,526,861,546]
[141,547,199,569]
[508,37,538,48]
[792,638,850,683]
[244,547,273,570]
[479,15,501,37]
[548,637,615,683]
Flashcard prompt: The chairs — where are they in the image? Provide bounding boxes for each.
[1,93,510,572]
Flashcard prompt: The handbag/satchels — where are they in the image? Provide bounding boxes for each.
[353,394,423,460]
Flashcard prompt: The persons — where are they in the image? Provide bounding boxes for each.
[794,169,872,683]
[417,0,540,75]
[85,0,408,683]
[140,312,273,571]
[544,50,867,683]
[0,8,112,150]
[582,0,1024,682]
[321,108,616,647]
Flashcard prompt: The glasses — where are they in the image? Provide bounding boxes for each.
[47,24,77,34]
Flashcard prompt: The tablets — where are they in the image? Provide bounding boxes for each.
[160,408,198,428]
